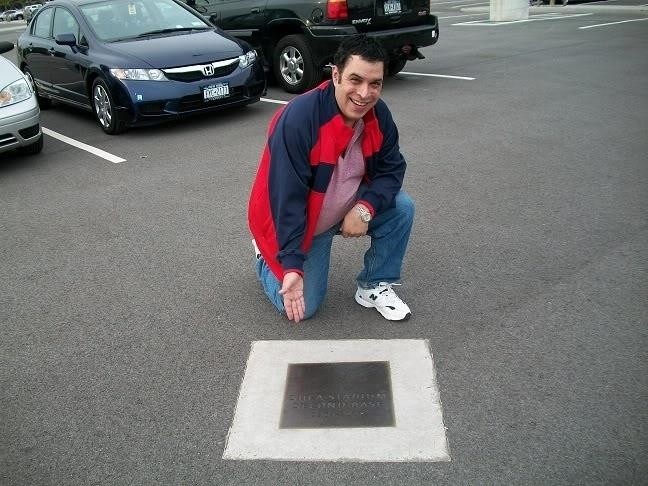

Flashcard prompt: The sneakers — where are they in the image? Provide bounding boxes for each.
[355,283,411,321]
[251,240,262,260]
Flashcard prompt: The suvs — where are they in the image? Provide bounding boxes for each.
[182,0,439,94]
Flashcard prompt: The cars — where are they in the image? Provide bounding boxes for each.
[14,0,267,134]
[0,1,46,24]
[0,37,44,160]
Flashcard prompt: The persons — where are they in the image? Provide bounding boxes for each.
[249,33,414,322]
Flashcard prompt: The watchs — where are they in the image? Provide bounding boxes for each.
[355,205,372,223]
